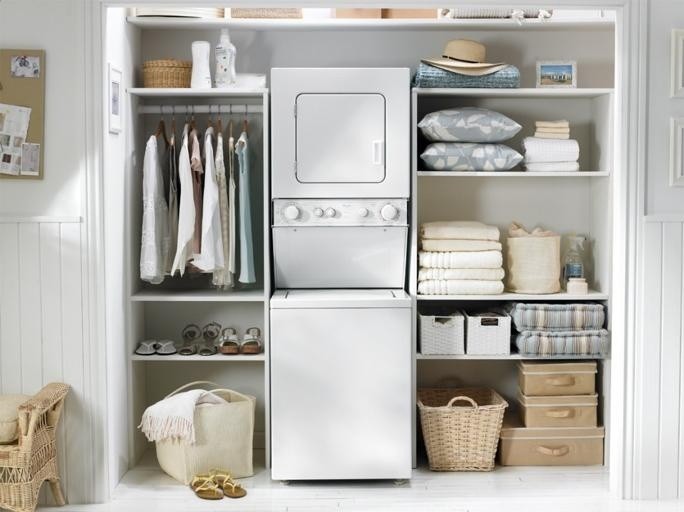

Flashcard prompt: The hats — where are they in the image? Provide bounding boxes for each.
[419,38,508,78]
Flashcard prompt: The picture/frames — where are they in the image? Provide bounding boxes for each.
[106,63,125,133]
[534,61,579,88]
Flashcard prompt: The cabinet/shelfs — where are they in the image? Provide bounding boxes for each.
[124,86,270,482]
[410,85,615,477]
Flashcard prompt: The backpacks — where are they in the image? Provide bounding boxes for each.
[504,232,562,295]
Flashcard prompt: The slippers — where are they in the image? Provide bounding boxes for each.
[134,338,158,355]
[156,339,178,355]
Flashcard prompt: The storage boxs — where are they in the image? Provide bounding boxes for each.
[513,383,601,429]
[499,413,605,469]
[515,359,600,397]
[153,389,255,483]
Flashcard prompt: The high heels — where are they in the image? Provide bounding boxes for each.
[176,323,202,355]
[197,321,223,356]
[218,326,240,355]
[240,325,263,354]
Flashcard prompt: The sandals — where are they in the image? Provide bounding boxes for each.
[208,466,248,499]
[189,473,225,500]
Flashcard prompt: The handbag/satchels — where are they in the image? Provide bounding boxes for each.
[152,378,258,487]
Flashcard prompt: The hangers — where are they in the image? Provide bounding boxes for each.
[151,105,249,151]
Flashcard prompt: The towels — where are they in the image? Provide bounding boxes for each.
[521,117,582,172]
[419,214,506,297]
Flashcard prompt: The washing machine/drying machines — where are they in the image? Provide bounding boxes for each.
[267,67,415,483]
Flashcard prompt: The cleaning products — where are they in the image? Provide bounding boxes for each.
[561,235,587,290]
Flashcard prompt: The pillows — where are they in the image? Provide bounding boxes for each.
[0,388,36,445]
[418,107,523,144]
[420,140,523,172]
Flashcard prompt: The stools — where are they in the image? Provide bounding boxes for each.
[0,380,71,511]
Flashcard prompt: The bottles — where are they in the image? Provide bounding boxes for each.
[189,41,212,89]
[566,278,588,293]
[214,28,236,89]
